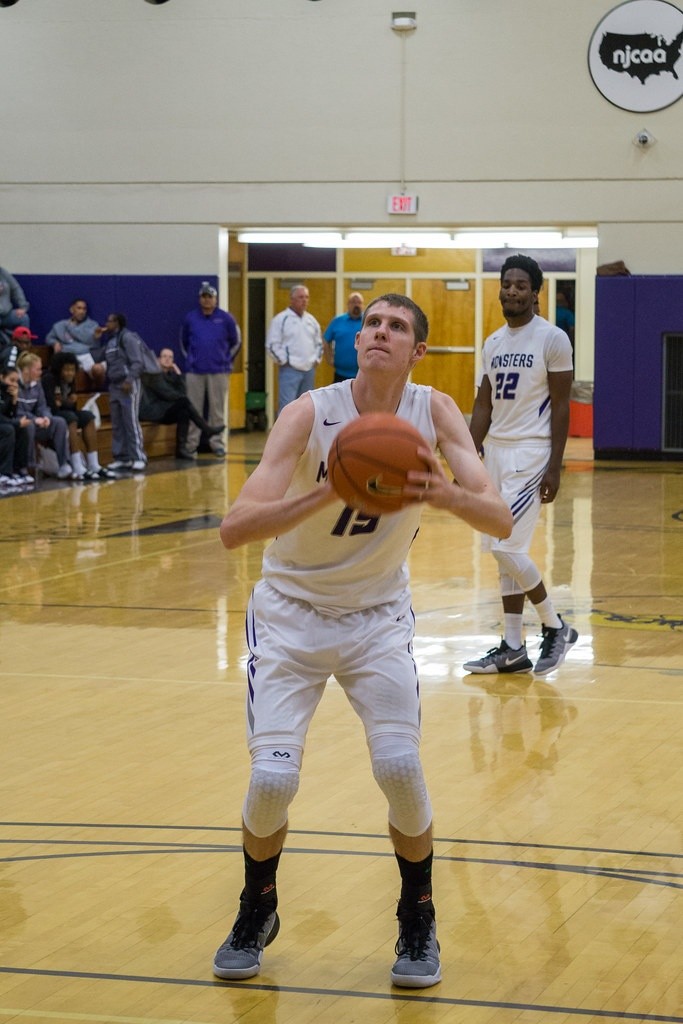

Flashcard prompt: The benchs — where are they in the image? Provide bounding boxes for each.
[32,343,179,465]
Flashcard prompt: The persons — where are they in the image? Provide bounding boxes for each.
[0,266,367,487]
[211,294,513,987]
[463,254,579,675]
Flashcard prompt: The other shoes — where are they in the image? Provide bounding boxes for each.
[204,424,225,439]
[108,459,145,471]
[215,448,226,457]
[57,470,85,481]
[83,467,117,481]
[175,452,194,460]
[0,467,35,486]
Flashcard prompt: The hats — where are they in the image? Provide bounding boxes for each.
[13,326,38,339]
[199,285,217,297]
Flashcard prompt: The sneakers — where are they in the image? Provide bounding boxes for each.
[463,634,534,674]
[534,613,578,676]
[213,900,280,978]
[391,899,443,988]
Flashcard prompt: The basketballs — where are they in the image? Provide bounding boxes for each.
[327,413,429,516]
